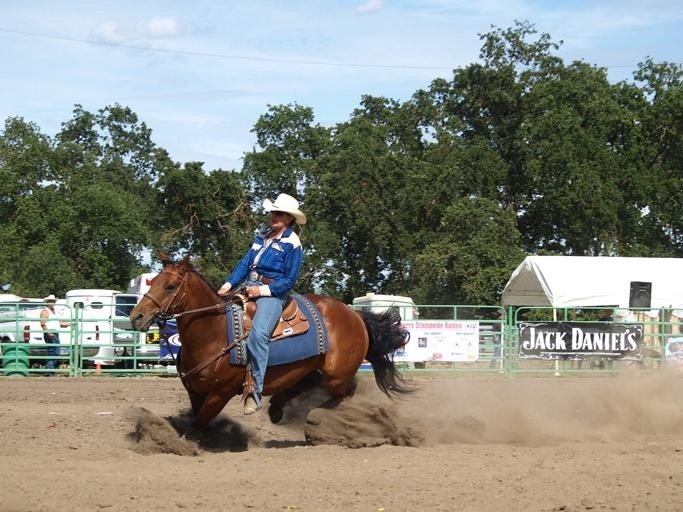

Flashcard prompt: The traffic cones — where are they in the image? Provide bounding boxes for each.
[93,362,104,375]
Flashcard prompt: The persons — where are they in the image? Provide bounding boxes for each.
[598,307,612,321]
[38,294,71,377]
[488,311,505,371]
[215,192,305,415]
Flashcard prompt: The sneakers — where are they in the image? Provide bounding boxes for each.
[243,392,262,415]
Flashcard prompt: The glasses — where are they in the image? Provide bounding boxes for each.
[274,211,293,216]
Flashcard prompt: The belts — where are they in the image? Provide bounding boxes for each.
[251,274,274,284]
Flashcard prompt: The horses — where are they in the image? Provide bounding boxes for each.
[129,249,418,428]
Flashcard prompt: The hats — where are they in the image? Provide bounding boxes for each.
[263,192,307,226]
[43,295,58,303]
